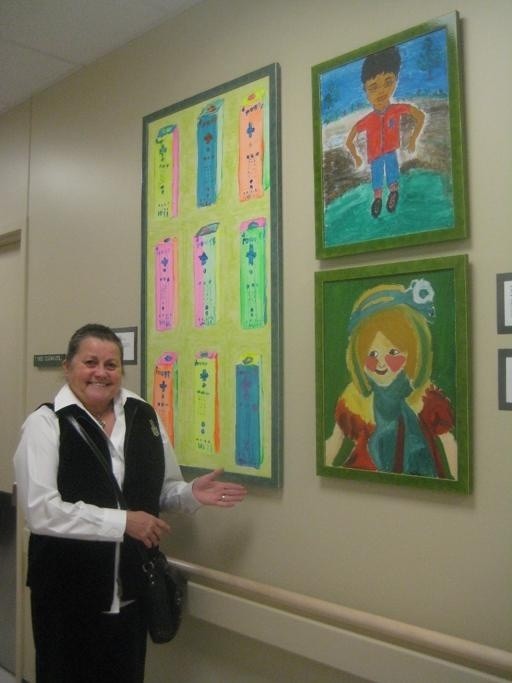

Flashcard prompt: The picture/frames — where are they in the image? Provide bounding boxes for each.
[314,253,471,495]
[138,61,284,490]
[497,272,512,334]
[113,325,139,366]
[497,349,510,409]
[313,11,471,262]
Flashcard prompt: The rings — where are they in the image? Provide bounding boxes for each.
[221,495,225,502]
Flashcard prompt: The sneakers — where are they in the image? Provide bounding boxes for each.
[386,192,398,210]
[371,198,382,218]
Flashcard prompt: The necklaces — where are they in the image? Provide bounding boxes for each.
[89,409,111,428]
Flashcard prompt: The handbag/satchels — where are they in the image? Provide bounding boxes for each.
[131,547,182,645]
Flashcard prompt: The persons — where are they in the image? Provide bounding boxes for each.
[345,45,426,217]
[12,323,248,683]
[325,281,458,481]
